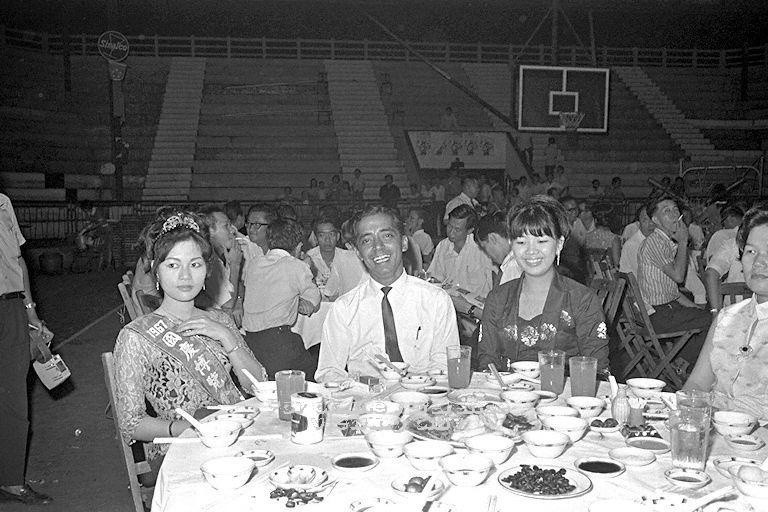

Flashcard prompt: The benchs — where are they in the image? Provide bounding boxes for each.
[1,42,767,208]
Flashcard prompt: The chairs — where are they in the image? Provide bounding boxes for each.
[104,352,149,512]
[116,266,156,324]
[574,248,758,391]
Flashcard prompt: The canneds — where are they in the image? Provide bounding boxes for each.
[289,391,325,446]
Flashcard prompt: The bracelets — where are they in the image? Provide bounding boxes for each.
[169,419,176,438]
[24,301,38,310]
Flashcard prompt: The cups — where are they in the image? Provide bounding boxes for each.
[538,347,597,395]
[446,345,473,388]
[668,390,714,472]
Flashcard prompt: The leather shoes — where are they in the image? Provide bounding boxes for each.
[2,484,50,505]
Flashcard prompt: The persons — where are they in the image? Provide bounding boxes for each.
[543,137,561,181]
[112,212,270,480]
[677,205,768,429]
[0,190,55,507]
[438,106,458,132]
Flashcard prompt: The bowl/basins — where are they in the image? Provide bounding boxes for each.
[667,471,711,489]
[712,408,767,512]
[194,359,667,509]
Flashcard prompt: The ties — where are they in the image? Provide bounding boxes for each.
[380,286,405,362]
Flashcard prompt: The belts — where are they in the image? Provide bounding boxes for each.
[0,291,26,301]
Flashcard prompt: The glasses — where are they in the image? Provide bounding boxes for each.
[245,222,268,229]
